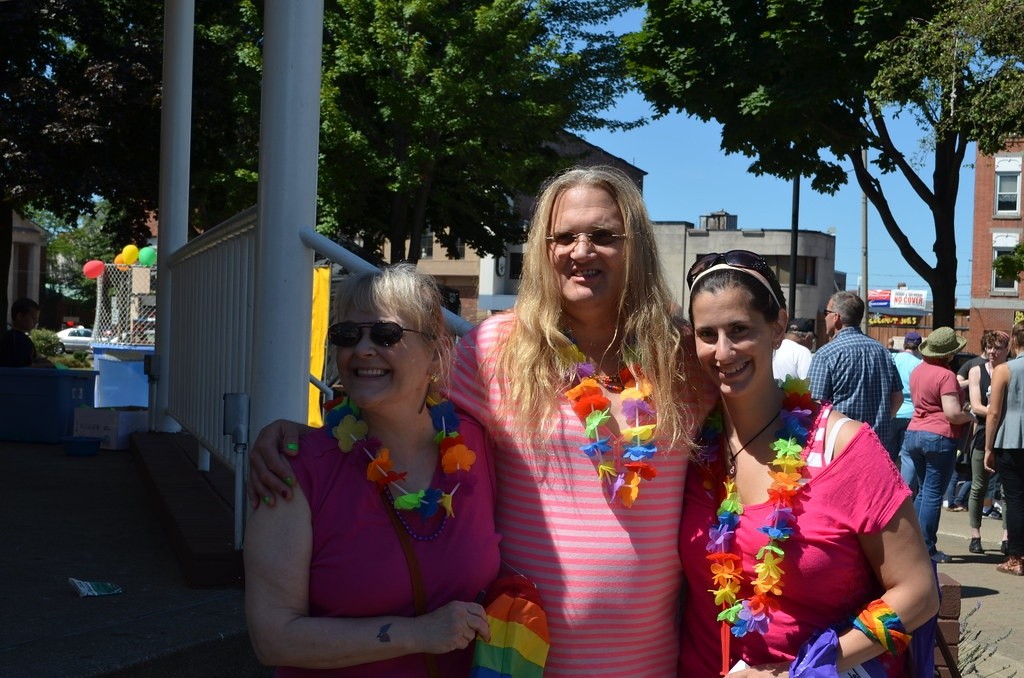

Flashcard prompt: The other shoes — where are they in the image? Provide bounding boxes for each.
[951,501,968,511]
[969,539,982,552]
[930,551,952,562]
[1001,540,1008,555]
[997,557,1024,575]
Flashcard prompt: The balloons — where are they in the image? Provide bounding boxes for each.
[114,244,157,272]
[83,259,104,279]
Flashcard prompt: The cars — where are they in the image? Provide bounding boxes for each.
[41,327,92,356]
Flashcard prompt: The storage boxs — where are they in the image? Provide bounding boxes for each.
[74,406,150,451]
[0,367,100,444]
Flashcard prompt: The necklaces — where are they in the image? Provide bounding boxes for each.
[322,393,477,524]
[557,324,657,507]
[699,372,818,638]
[380,486,449,543]
[721,386,787,481]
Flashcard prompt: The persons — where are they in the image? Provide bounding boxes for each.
[0,295,41,369]
[890,334,924,462]
[771,317,819,382]
[246,161,720,678]
[942,317,1024,576]
[678,250,939,678]
[244,262,503,678]
[899,326,978,563]
[803,290,905,460]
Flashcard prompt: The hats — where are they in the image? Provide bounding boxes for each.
[918,327,967,359]
[787,318,819,339]
[904,332,921,345]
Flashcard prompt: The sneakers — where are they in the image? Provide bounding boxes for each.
[981,505,1002,519]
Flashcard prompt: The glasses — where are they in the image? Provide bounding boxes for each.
[325,321,436,347]
[824,309,840,317]
[687,250,770,287]
[546,228,627,246]
